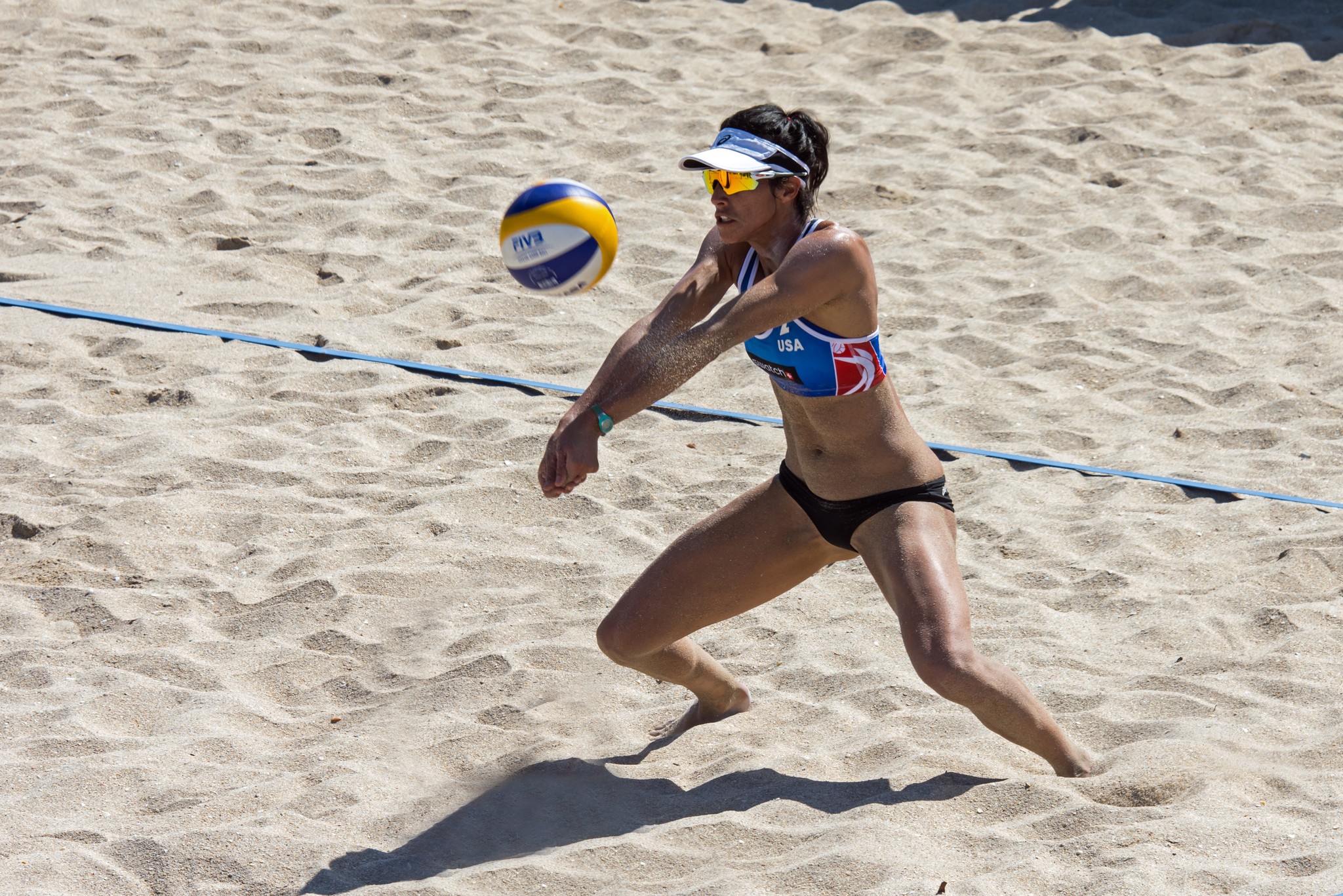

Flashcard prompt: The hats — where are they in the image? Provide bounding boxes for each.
[678,128,811,191]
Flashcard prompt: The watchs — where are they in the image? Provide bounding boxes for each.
[590,402,614,436]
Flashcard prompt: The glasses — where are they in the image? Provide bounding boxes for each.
[702,170,807,195]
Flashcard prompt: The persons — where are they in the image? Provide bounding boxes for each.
[538,103,1098,778]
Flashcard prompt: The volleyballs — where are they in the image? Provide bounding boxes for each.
[497,175,620,299]
